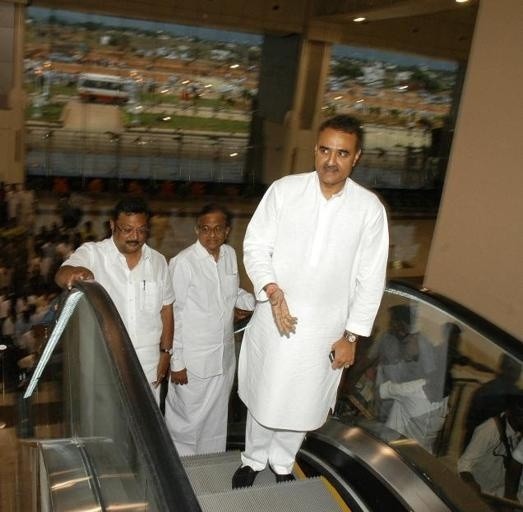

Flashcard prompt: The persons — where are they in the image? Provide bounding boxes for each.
[0,178,113,383]
[232,114,390,489]
[165,205,256,458]
[352,304,523,511]
[55,197,175,408]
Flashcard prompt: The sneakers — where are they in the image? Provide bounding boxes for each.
[232,463,264,490]
[268,464,296,483]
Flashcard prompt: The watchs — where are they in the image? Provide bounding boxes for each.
[344,332,360,344]
[160,347,173,356]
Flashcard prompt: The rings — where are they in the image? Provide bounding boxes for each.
[344,363,350,369]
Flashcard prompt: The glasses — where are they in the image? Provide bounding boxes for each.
[114,224,148,233]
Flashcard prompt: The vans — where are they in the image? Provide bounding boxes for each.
[78,72,130,105]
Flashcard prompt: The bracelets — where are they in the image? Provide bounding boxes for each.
[266,285,276,298]
